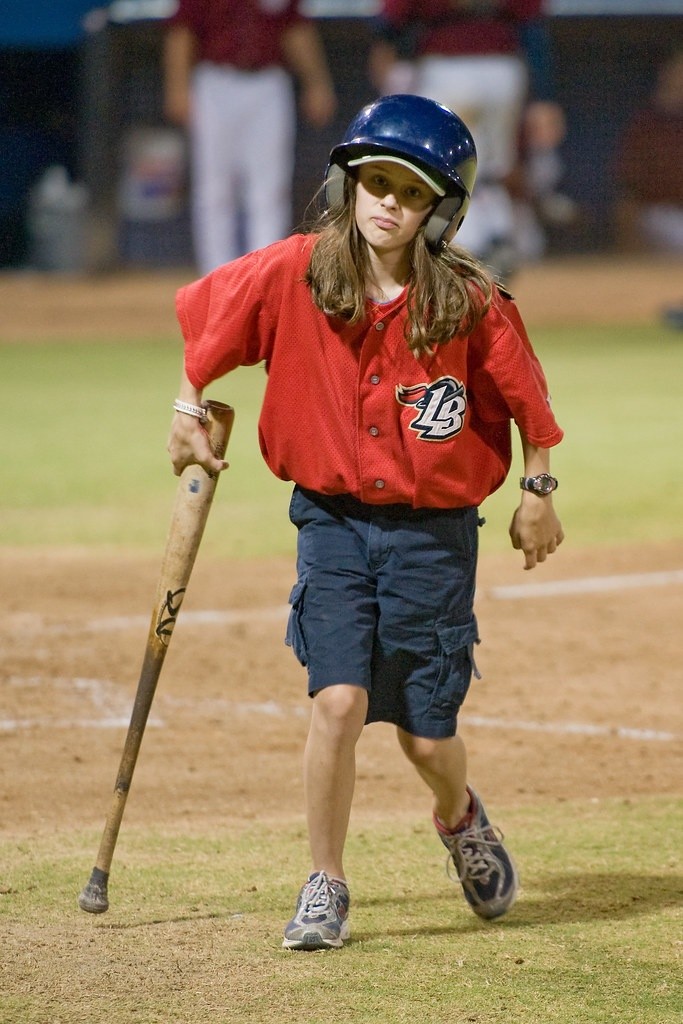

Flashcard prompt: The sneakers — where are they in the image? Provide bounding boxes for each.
[282,870,351,951]
[431,782,517,921]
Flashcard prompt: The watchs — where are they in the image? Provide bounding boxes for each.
[520,473,558,495]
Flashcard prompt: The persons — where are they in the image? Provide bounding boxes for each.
[622,57,683,327]
[166,93,564,950]
[165,0,335,278]
[367,0,544,260]
[0,0,116,267]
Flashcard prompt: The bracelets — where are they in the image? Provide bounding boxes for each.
[173,399,206,423]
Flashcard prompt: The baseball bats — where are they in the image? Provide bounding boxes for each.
[77,399,236,914]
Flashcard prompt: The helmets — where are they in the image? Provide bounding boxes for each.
[324,94,477,255]
